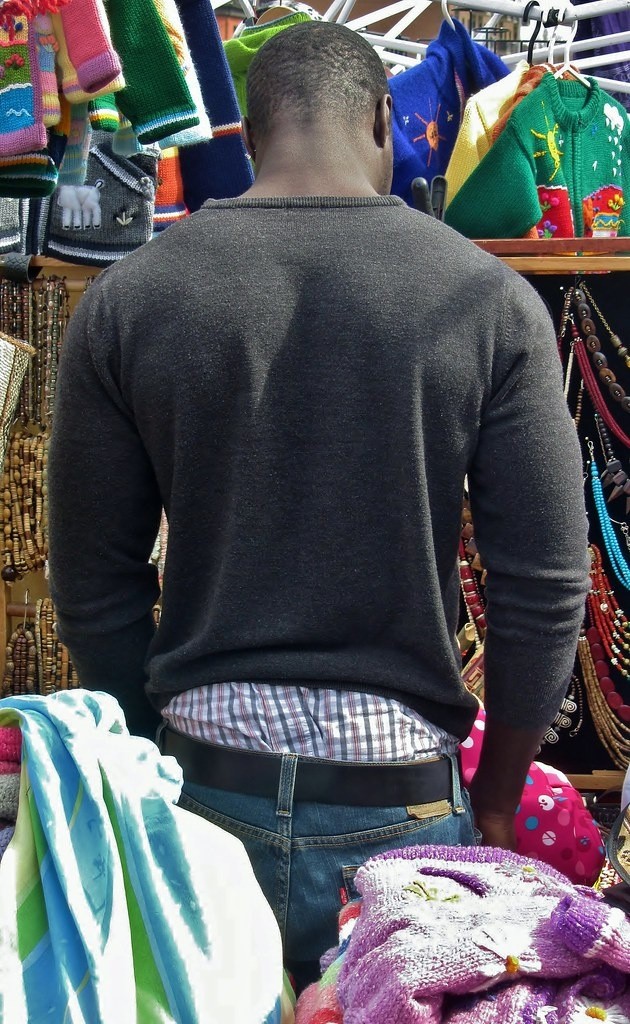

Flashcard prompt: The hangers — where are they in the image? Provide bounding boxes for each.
[216,0,313,52]
[387,0,627,122]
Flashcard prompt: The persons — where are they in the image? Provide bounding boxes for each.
[47,21,592,1000]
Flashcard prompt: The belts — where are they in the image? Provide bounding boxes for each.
[150,715,464,808]
